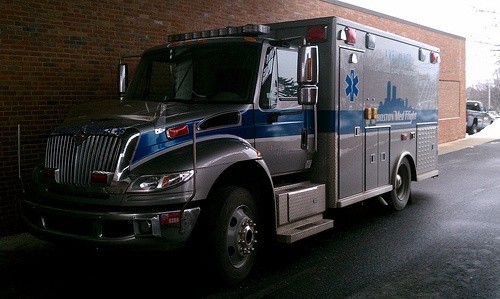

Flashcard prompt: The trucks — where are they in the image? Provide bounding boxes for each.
[21,16,439,283]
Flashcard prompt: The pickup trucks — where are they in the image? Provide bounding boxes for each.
[467,101,495,135]
